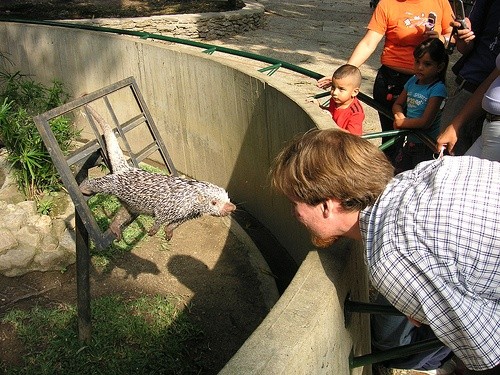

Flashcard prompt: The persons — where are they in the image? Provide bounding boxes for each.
[316,0,500,375]
[266,128,500,375]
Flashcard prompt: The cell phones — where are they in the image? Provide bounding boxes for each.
[424,12,437,31]
[456,19,466,30]
